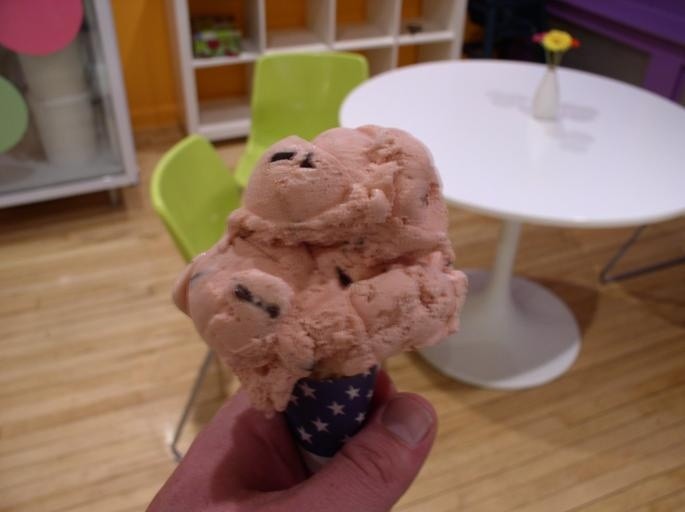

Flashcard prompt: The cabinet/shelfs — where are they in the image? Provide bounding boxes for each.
[164,0,469,144]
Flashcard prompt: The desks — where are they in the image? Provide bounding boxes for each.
[337,57,685,390]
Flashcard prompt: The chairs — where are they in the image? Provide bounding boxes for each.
[231,49,372,193]
[150,135,252,450]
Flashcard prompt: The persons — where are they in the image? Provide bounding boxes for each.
[141,359,441,511]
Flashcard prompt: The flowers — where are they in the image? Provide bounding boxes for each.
[533,29,579,71]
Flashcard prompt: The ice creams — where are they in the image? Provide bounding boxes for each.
[173,125,468,474]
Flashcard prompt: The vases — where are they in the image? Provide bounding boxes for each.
[532,71,562,120]
